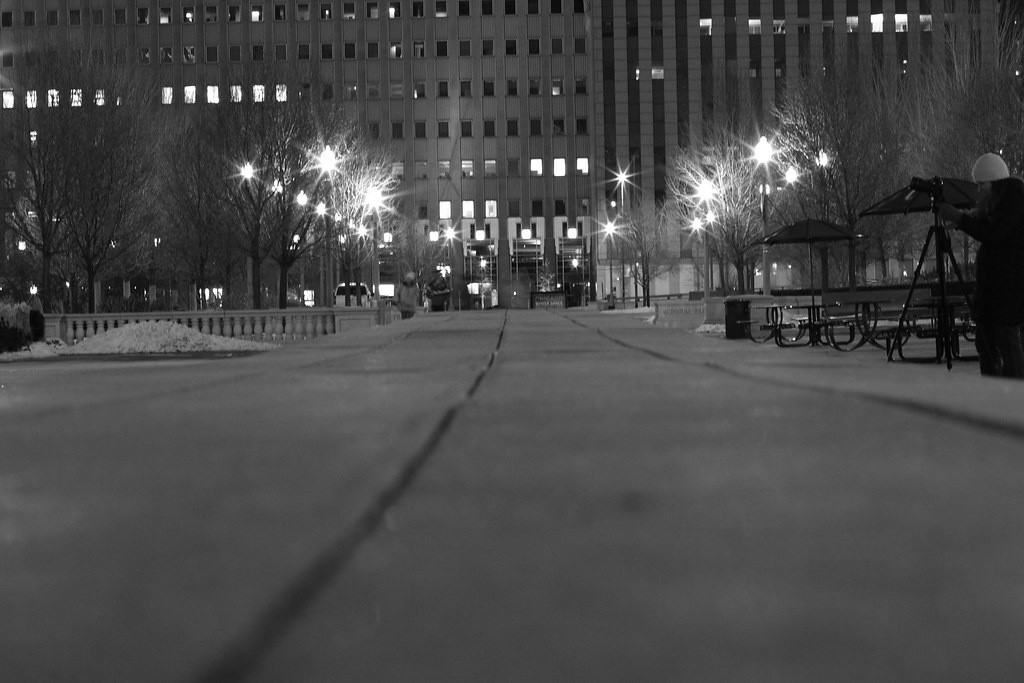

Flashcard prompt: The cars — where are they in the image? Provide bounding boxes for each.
[332,281,374,309]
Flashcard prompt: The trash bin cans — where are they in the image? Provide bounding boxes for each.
[723,294,751,341]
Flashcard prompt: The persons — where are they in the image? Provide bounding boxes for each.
[425,269,454,312]
[394,272,420,320]
[930,153,1024,381]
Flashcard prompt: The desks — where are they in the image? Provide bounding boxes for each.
[785,304,836,348]
[904,297,968,364]
[756,304,796,348]
[835,298,895,351]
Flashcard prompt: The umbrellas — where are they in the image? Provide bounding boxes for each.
[858,175,981,217]
[751,217,869,305]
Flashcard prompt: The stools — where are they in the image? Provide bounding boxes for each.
[735,318,977,371]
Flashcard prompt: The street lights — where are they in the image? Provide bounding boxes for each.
[604,222,616,309]
[319,145,336,308]
[754,136,772,297]
[444,227,455,312]
[366,186,382,298]
[617,172,628,308]
[296,190,308,297]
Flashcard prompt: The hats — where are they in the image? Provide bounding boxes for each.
[406,273,415,281]
